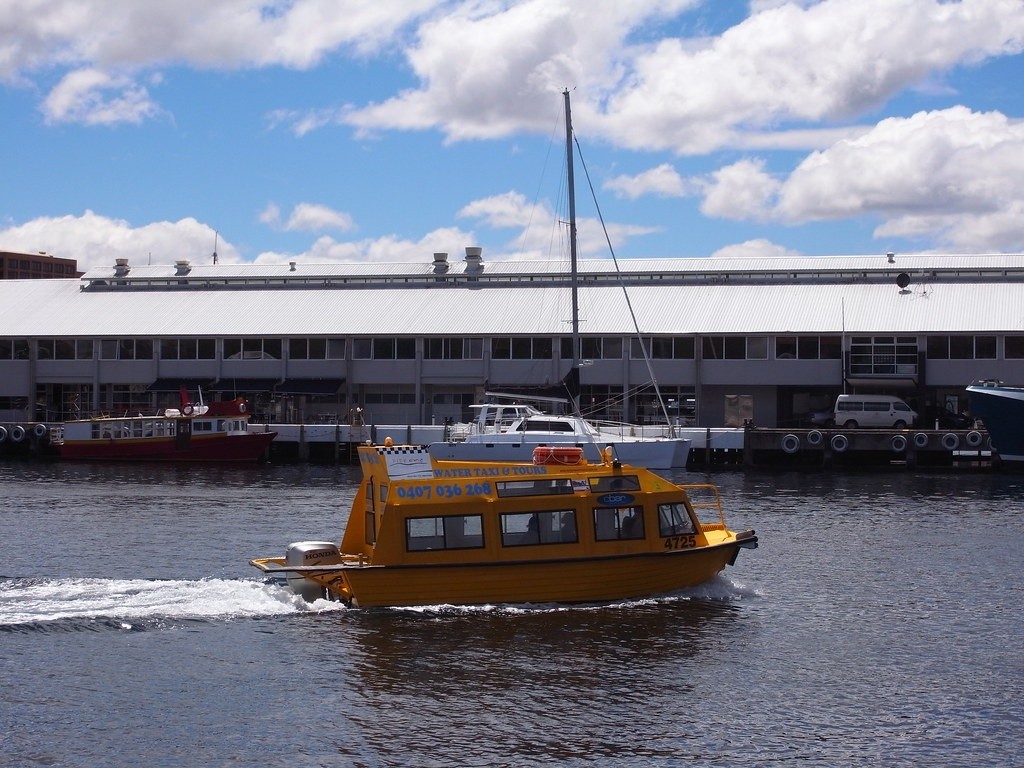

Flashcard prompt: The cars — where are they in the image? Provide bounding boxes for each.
[925,405,972,428]
[807,406,836,428]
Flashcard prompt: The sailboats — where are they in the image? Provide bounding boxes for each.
[426,87,695,471]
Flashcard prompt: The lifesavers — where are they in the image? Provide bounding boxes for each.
[831,435,848,452]
[182,402,194,416]
[534,446,583,456]
[890,435,908,454]
[913,433,929,448]
[0,426,7,443]
[941,433,960,450]
[966,431,982,447]
[806,430,824,446]
[780,434,800,454]
[33,423,47,438]
[240,403,246,413]
[8,425,25,444]
[533,456,584,465]
[987,437,998,452]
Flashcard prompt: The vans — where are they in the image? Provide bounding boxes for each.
[831,393,919,429]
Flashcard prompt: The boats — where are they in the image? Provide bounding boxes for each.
[54,380,278,463]
[249,433,766,611]
[965,377,1024,474]
